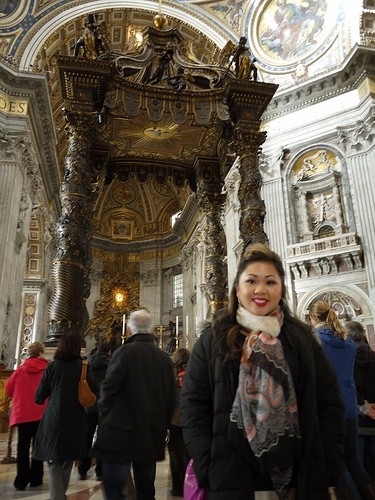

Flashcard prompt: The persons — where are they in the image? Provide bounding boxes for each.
[76,338,113,482]
[5,341,53,492]
[167,345,193,499]
[97,307,178,500]
[35,331,99,500]
[307,299,372,499]
[178,243,343,500]
[343,321,374,499]
